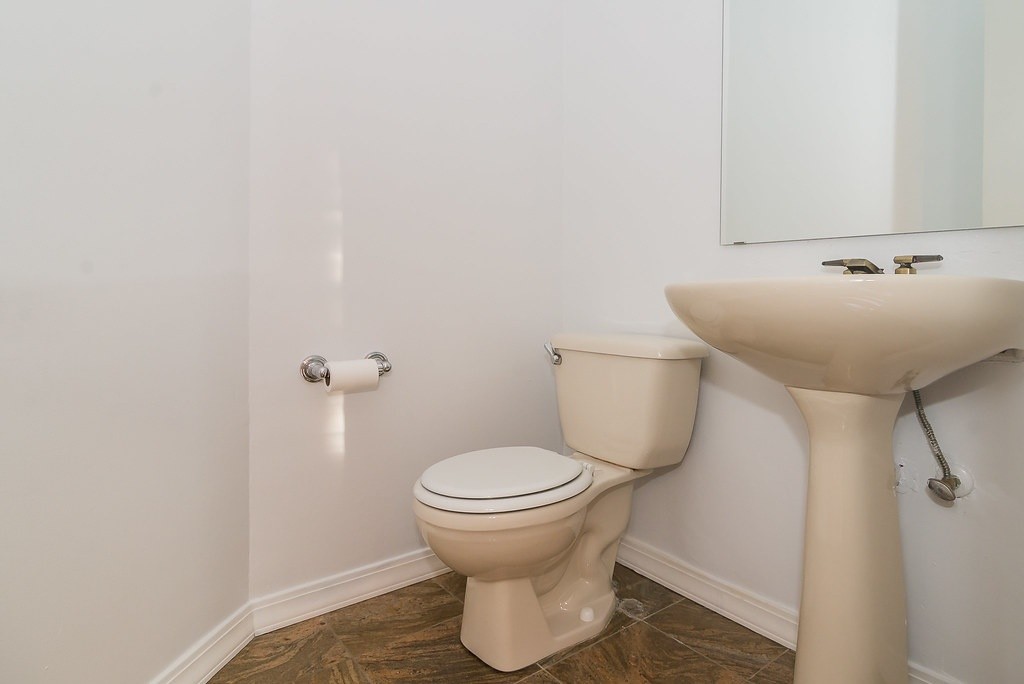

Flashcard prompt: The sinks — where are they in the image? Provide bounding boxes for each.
[663,272,1024,397]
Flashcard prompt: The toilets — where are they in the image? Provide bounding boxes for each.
[409,329,711,674]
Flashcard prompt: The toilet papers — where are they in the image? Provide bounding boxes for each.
[322,359,379,394]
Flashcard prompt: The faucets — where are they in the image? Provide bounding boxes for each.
[842,258,885,276]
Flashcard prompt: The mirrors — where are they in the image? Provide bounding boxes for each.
[721,1,1024,252]
